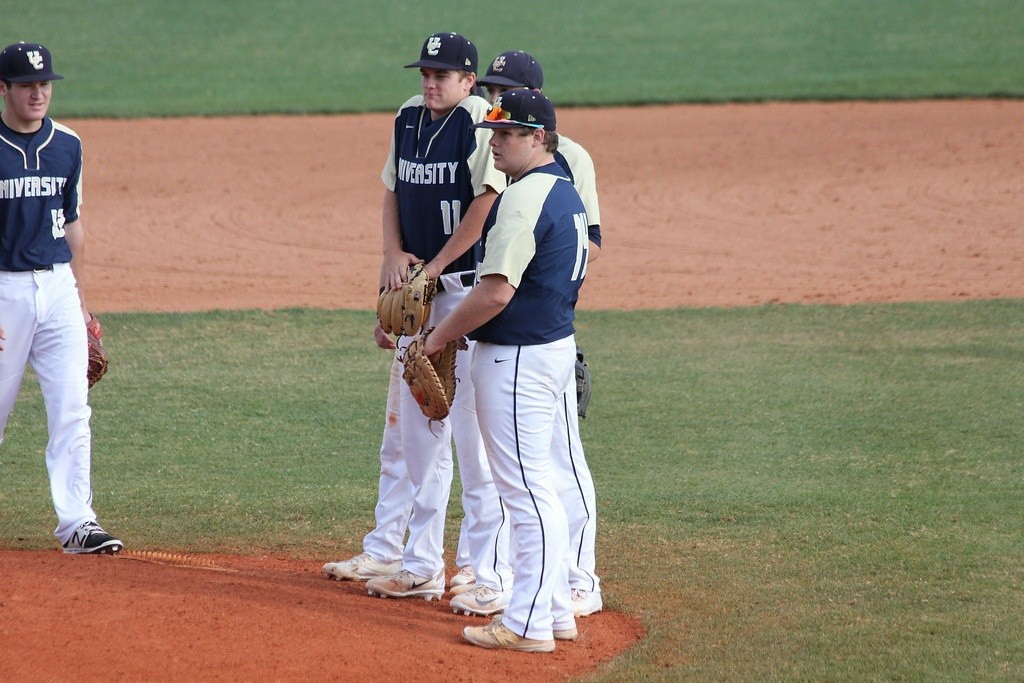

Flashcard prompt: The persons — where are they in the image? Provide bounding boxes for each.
[0,43,123,555]
[320,29,603,652]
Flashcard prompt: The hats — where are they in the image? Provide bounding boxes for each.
[0,41,64,83]
[404,32,478,76]
[476,50,543,89]
[468,86,556,131]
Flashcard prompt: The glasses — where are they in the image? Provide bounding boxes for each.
[480,106,546,130]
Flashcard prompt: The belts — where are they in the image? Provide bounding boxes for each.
[3,261,70,272]
[436,272,476,293]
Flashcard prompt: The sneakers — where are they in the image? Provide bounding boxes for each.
[553,628,578,640]
[63,521,123,556]
[570,588,603,618]
[449,579,477,599]
[322,553,402,582]
[462,618,555,653]
[449,585,514,617]
[450,566,477,587]
[365,565,445,602]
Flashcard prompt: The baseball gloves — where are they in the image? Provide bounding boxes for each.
[402,326,469,438]
[377,263,438,337]
[87,312,109,389]
